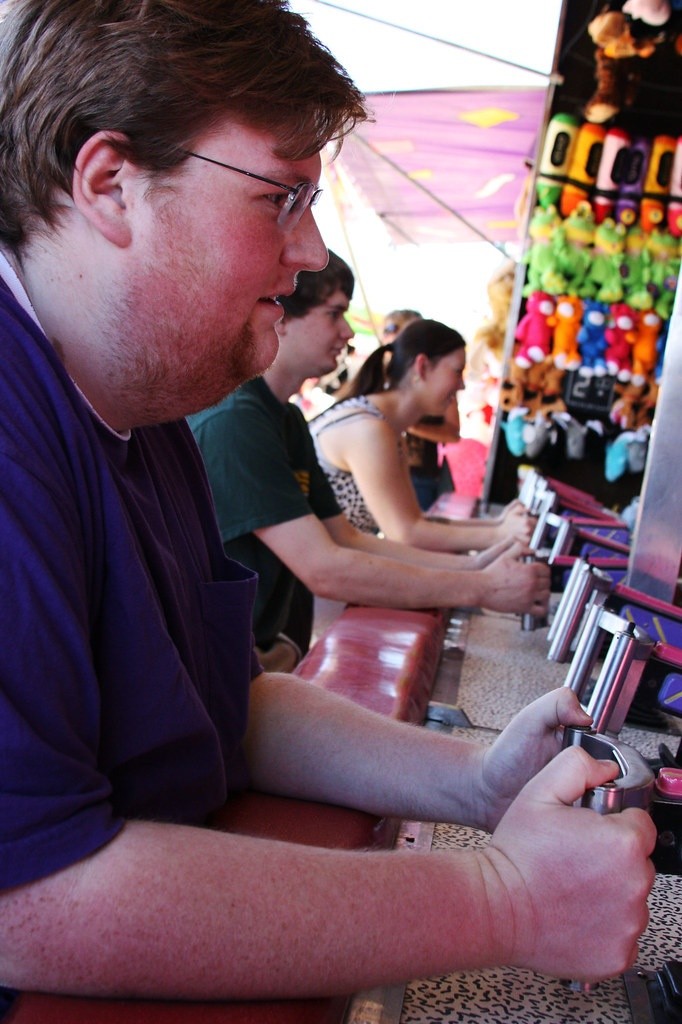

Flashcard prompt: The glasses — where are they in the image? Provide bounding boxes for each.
[383,324,398,333]
[162,142,324,231]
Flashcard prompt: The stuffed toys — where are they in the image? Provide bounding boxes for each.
[501,0,682,482]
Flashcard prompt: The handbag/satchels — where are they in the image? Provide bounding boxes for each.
[434,454,455,495]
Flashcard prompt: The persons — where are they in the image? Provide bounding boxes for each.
[0,0,655,996]
[338,310,460,509]
[307,319,536,549]
[181,249,548,668]
